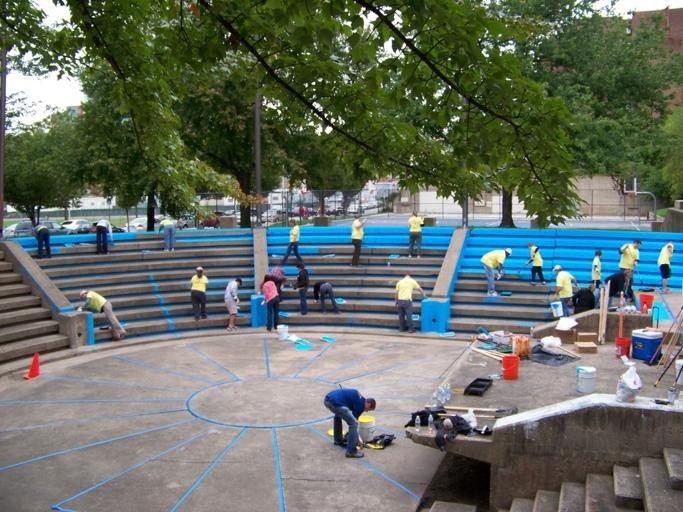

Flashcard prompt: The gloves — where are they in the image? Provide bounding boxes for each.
[362,219,367,224]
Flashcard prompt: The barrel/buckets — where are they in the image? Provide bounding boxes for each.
[276,324,289,341]
[577,367,596,394]
[675,360,683,385]
[550,301,564,318]
[614,338,631,359]
[640,295,653,312]
[356,414,376,441]
[501,356,520,380]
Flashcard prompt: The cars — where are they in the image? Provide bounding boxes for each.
[124,217,161,232]
[60,220,89,233]
[197,202,381,229]
[78,221,124,233]
[173,214,195,231]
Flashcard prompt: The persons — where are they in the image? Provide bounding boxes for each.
[281,220,304,268]
[78,290,126,342]
[263,275,280,331]
[526,242,547,285]
[659,241,675,294]
[95,219,113,255]
[258,267,287,302]
[395,272,426,334]
[31,224,52,258]
[225,278,242,331]
[480,248,512,296]
[408,210,424,259]
[158,217,175,252]
[351,213,367,267]
[190,266,208,321]
[551,237,643,316]
[313,281,340,314]
[293,263,310,314]
[324,388,375,458]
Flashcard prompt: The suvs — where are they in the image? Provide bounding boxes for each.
[8,220,76,237]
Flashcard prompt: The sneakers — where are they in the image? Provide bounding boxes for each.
[488,291,497,294]
[346,452,364,457]
[663,288,671,293]
[541,282,546,285]
[488,293,497,296]
[335,439,349,444]
[408,329,416,333]
[531,282,536,285]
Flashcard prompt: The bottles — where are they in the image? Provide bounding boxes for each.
[414,416,420,432]
[678,391,683,409]
[388,261,390,268]
[428,415,433,430]
[432,384,451,407]
[643,304,647,314]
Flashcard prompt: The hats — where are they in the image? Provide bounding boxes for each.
[196,267,203,271]
[505,248,512,254]
[80,291,87,301]
[552,265,561,271]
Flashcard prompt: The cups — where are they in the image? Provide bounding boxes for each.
[648,309,652,315]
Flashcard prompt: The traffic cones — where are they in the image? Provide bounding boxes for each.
[22,352,39,380]
